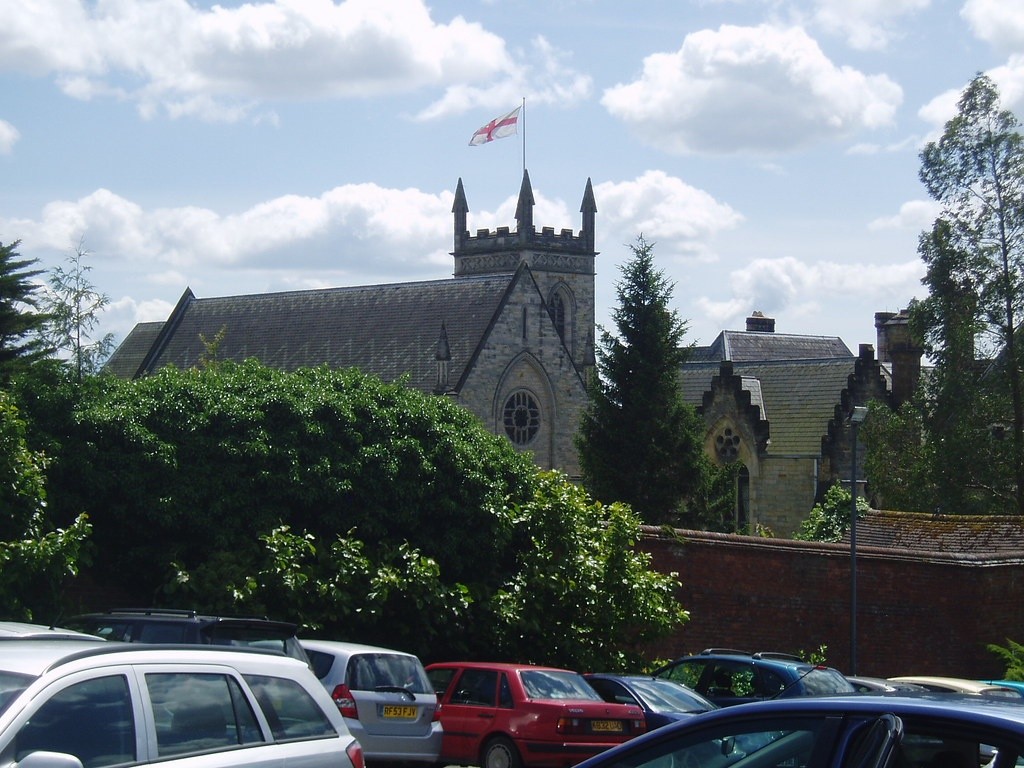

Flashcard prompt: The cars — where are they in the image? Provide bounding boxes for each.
[570,696,1024,768]
[0,620,106,642]
[425,661,721,768]
[845,676,1024,696]
[0,634,366,768]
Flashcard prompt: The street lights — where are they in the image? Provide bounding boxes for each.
[848,405,869,677]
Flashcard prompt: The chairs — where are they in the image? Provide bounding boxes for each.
[1,691,226,765]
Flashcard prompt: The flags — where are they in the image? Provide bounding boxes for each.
[468,106,522,147]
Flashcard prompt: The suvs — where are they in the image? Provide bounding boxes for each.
[60,608,314,675]
[647,648,857,708]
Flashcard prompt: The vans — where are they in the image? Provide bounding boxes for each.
[296,639,444,762]
